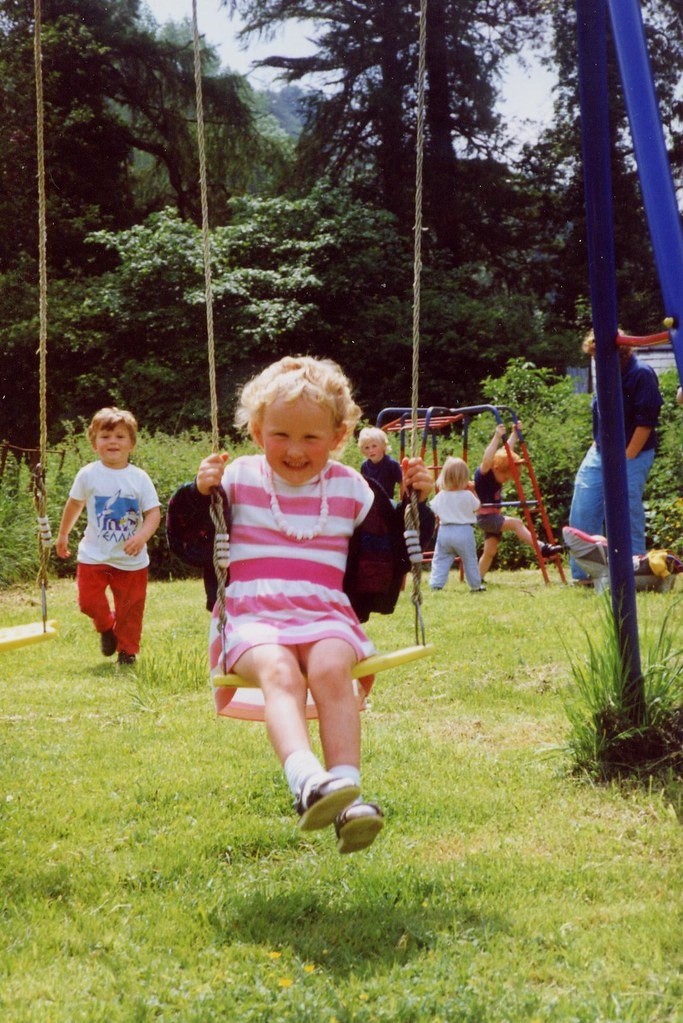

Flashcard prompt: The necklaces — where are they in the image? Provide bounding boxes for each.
[261,456,329,541]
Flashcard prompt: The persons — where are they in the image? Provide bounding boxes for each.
[428,457,488,592]
[166,355,440,856]
[357,426,405,499]
[474,419,563,586]
[566,326,666,588]
[54,404,165,665]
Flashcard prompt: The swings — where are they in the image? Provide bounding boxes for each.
[190,2,437,688]
[0,1,56,649]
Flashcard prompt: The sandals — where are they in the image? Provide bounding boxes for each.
[293,773,360,831]
[336,802,385,854]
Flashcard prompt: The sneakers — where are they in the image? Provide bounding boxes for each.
[540,544,569,556]
[100,630,116,655]
[117,651,135,664]
